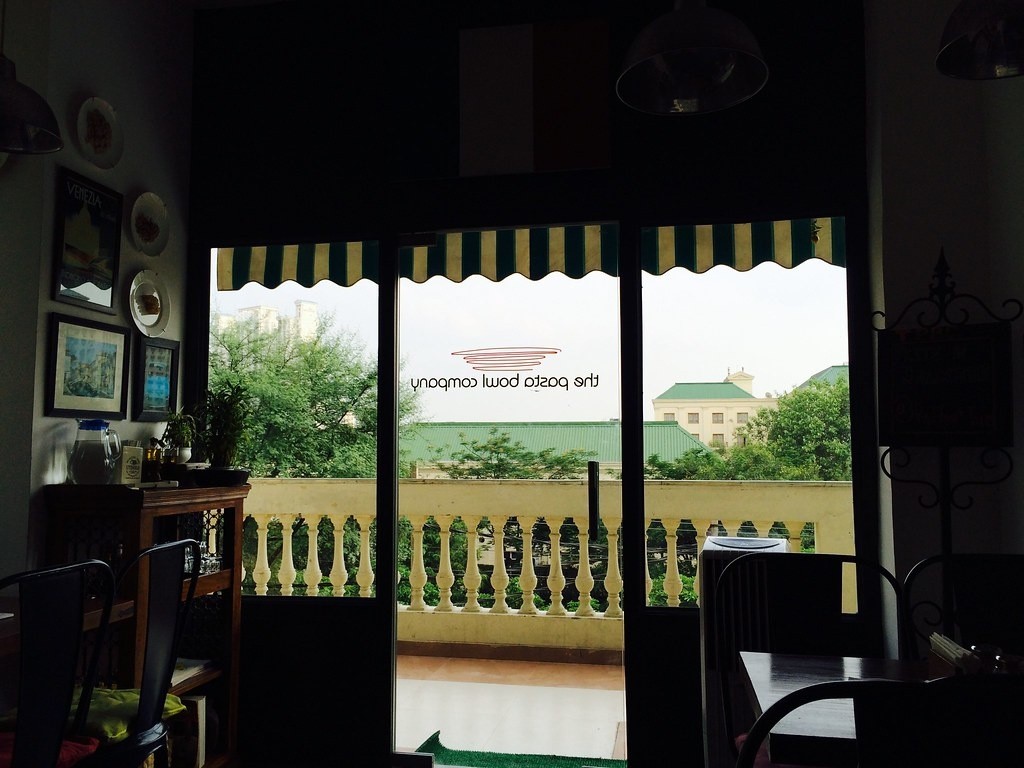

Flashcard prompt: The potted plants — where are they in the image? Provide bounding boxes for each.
[190,378,252,488]
[160,406,197,489]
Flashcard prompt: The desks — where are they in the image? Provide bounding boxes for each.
[0,596,135,683]
[732,650,1024,768]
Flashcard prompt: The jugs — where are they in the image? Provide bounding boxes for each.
[66,419,122,485]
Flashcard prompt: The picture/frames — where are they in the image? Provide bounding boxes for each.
[42,312,131,421]
[877,323,1015,450]
[130,336,180,423]
[50,164,124,317]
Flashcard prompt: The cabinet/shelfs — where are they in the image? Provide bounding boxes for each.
[44,483,251,768]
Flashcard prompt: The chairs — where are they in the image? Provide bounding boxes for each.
[0,559,117,768]
[736,679,1024,768]
[68,539,201,768]
[714,551,909,762]
[900,553,1024,658]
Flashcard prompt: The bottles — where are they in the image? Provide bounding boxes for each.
[142,447,176,483]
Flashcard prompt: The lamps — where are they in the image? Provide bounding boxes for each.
[0,52,65,155]
[615,0,769,116]
[935,0,1024,81]
